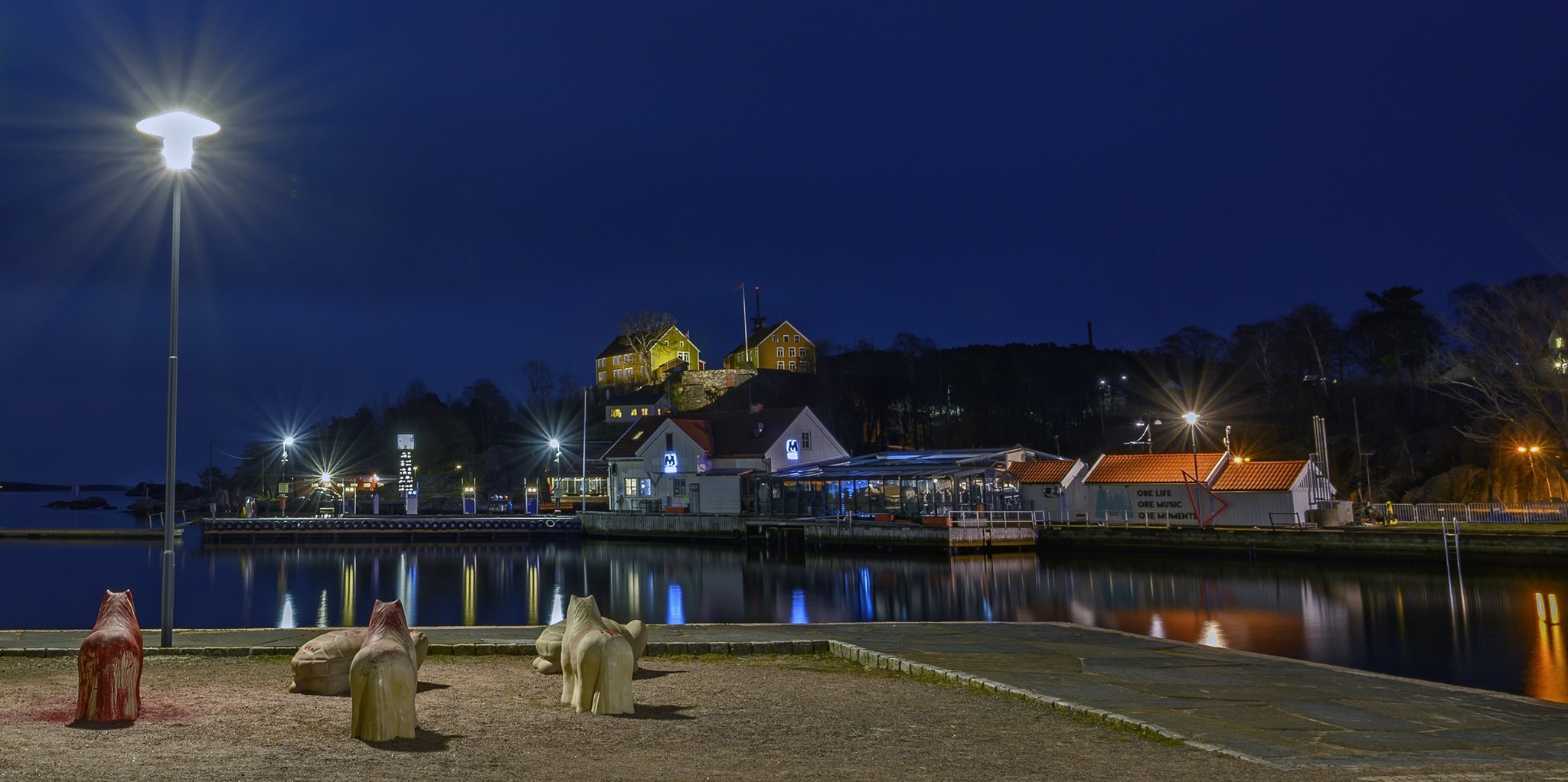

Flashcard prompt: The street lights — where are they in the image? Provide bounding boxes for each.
[1517,447,1543,501]
[134,109,223,649]
[1181,411,1203,529]
[549,439,560,510]
[282,436,294,517]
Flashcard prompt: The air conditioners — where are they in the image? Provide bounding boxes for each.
[1043,486,1054,496]
